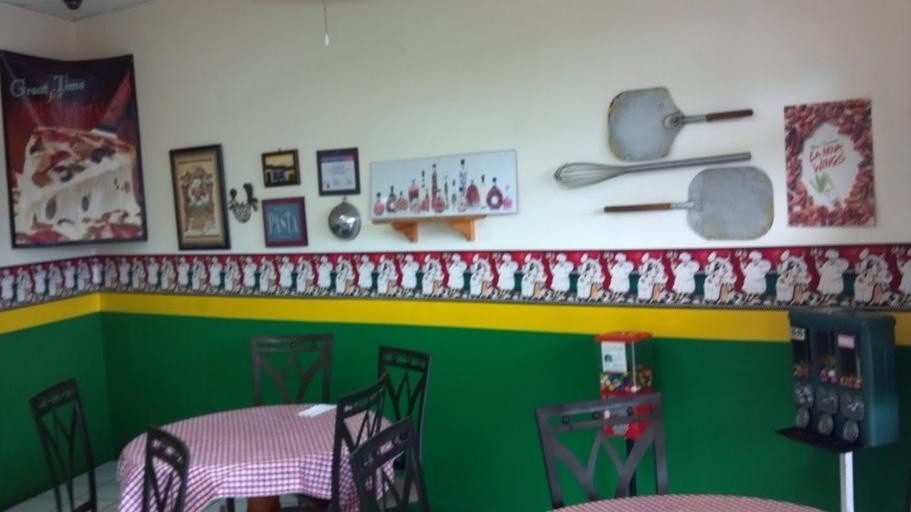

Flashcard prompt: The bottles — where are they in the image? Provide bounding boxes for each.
[373,159,514,215]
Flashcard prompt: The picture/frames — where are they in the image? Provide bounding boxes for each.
[315,147,360,196]
[261,197,308,247]
[261,149,301,188]
[169,143,232,250]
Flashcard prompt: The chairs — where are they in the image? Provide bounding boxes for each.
[349,416,430,512]
[28,377,98,511]
[250,333,334,406]
[295,371,414,512]
[534,392,668,509]
[140,421,190,512]
[377,343,431,480]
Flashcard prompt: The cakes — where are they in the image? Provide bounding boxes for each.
[12,126,143,246]
